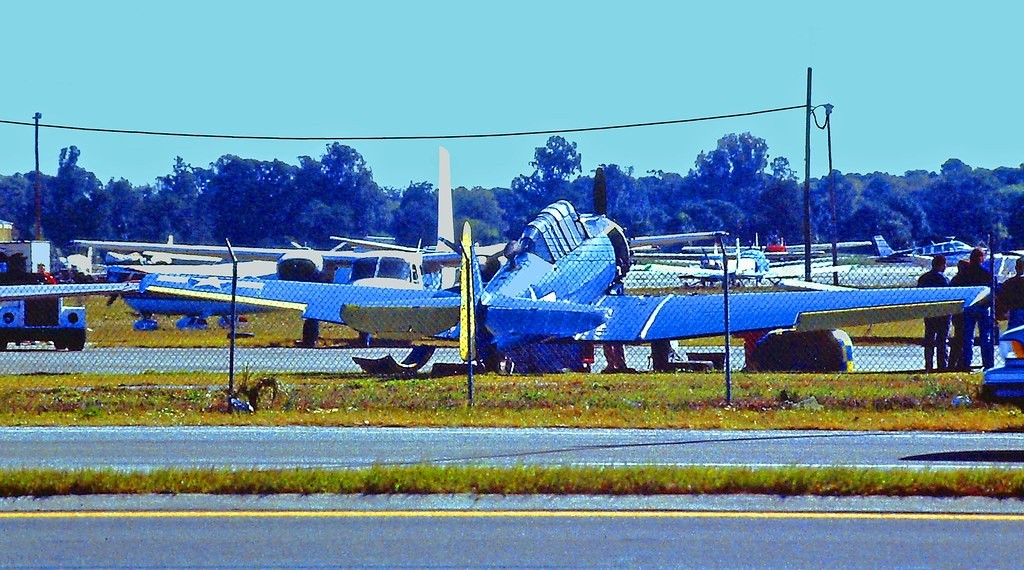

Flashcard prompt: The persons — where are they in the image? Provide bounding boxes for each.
[916,255,955,373]
[958,248,996,371]
[35,264,57,345]
[996,258,1024,332]
[948,260,971,371]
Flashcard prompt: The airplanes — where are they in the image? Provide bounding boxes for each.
[0,145,991,375]
[137,163,1023,374]
